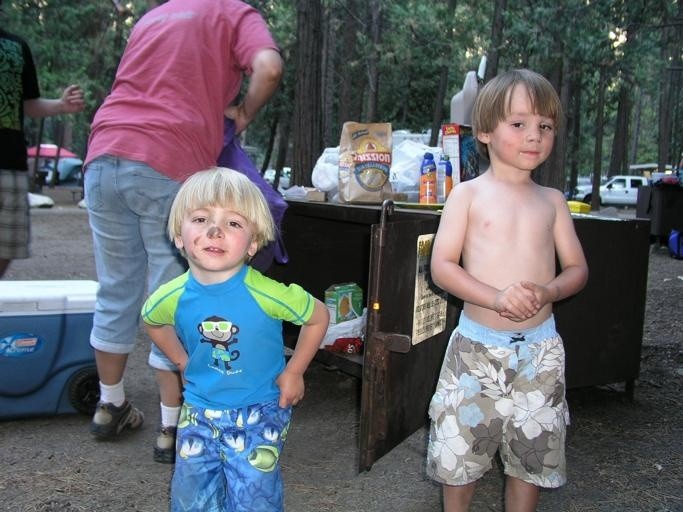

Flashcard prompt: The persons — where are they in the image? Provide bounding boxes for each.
[0,30,86,281]
[83,0,282,463]
[425,68,590,512]
[145,166,330,512]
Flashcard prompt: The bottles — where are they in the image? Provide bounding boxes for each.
[418,151,453,204]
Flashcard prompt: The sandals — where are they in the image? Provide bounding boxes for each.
[154,427,179,464]
[89,401,146,438]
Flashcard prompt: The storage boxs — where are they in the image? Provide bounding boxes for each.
[0,278,109,420]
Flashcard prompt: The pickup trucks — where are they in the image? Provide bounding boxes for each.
[571,174,652,209]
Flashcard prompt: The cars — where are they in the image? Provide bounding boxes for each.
[260,167,295,190]
[44,157,86,186]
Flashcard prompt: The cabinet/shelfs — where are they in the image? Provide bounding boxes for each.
[556,204,652,411]
[635,182,683,249]
[279,187,464,475]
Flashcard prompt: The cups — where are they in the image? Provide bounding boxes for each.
[651,173,664,184]
